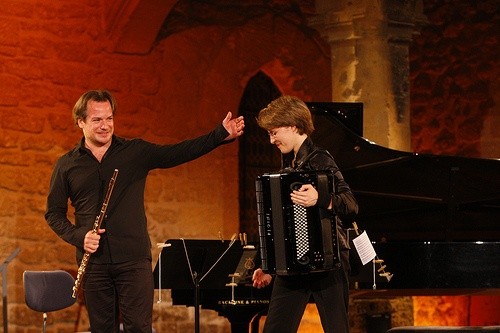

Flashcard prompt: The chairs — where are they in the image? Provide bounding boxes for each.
[22,270,76,333]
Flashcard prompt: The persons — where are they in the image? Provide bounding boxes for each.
[44,90,245,333]
[253,95,360,333]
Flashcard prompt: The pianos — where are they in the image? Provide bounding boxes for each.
[201,106,500,333]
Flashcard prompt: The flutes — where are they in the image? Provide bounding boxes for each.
[72,169,118,298]
